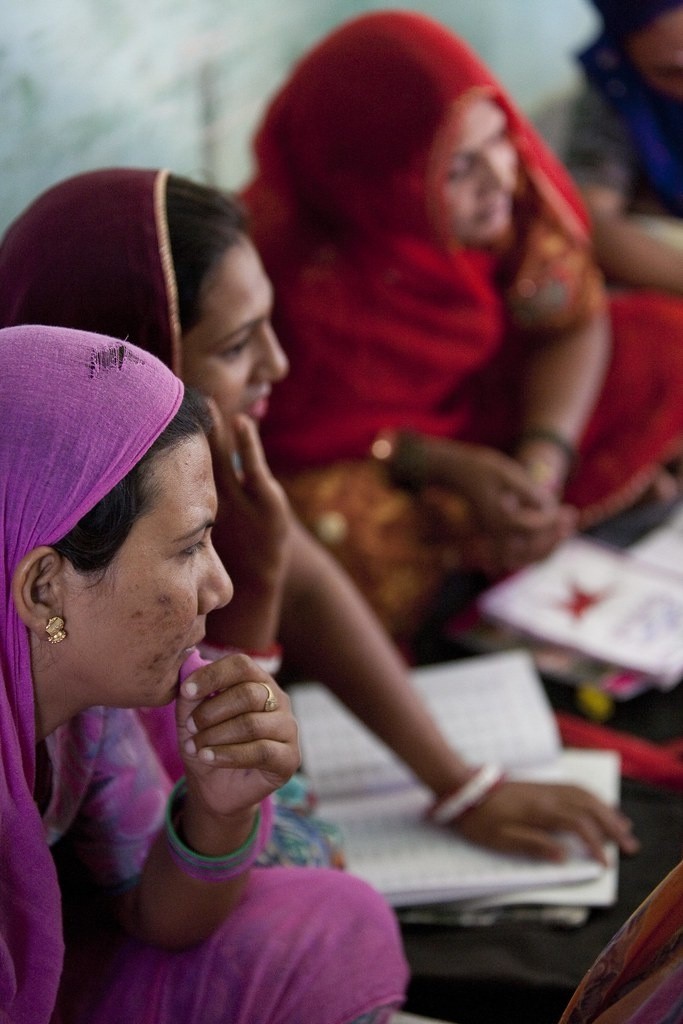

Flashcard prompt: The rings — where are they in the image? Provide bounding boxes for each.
[260,683,280,712]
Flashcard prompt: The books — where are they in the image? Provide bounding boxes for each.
[472,501,683,691]
[281,645,623,927]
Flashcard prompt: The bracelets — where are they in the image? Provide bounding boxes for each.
[423,761,508,828]
[503,423,579,472]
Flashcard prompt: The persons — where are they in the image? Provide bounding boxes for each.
[563,0,683,294]
[237,11,683,634]
[0,324,410,1024]
[0,164,640,870]
